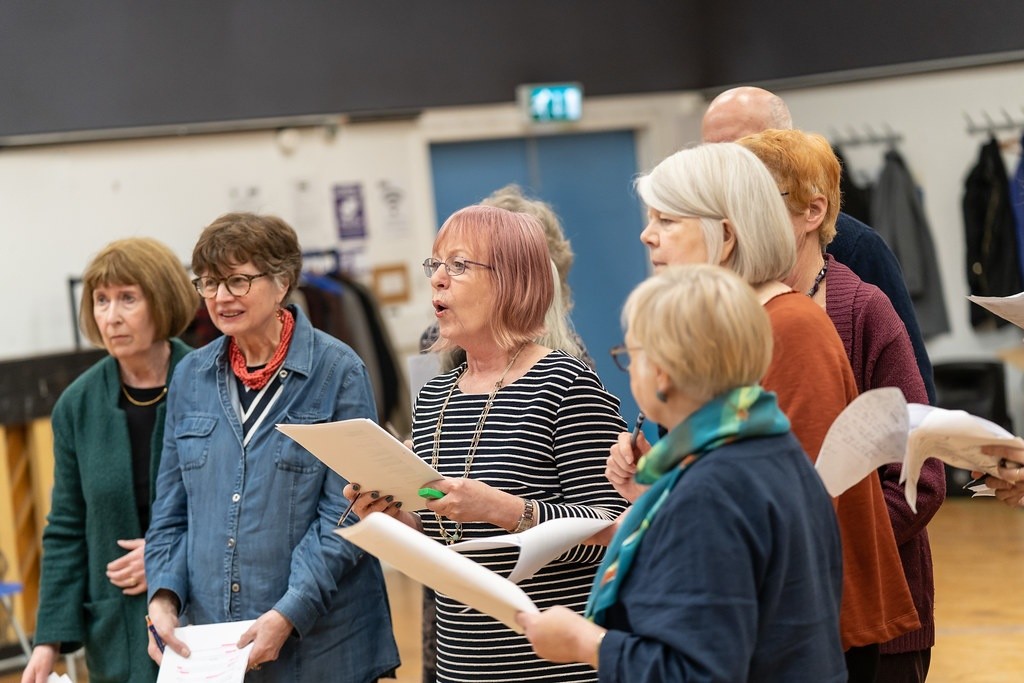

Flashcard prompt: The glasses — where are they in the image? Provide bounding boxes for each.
[423,256,495,277]
[610,343,644,373]
[191,272,269,298]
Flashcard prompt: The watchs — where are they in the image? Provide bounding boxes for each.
[506,498,534,534]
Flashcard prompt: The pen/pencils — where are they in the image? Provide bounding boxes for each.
[962,472,992,490]
[145,615,164,654]
[630,411,645,450]
[337,492,362,527]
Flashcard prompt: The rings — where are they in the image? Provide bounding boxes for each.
[1016,468,1022,481]
[130,576,137,586]
[254,662,258,667]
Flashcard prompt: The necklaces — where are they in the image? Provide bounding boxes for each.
[119,382,167,407]
[431,340,527,547]
[805,258,829,298]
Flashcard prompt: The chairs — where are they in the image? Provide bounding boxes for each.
[0,582,32,660]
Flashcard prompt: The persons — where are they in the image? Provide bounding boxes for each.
[343,190,632,683]
[19,210,402,683]
[512,86,946,683]
[971,443,1024,508]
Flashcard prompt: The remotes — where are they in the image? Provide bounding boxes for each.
[417,488,445,501]
